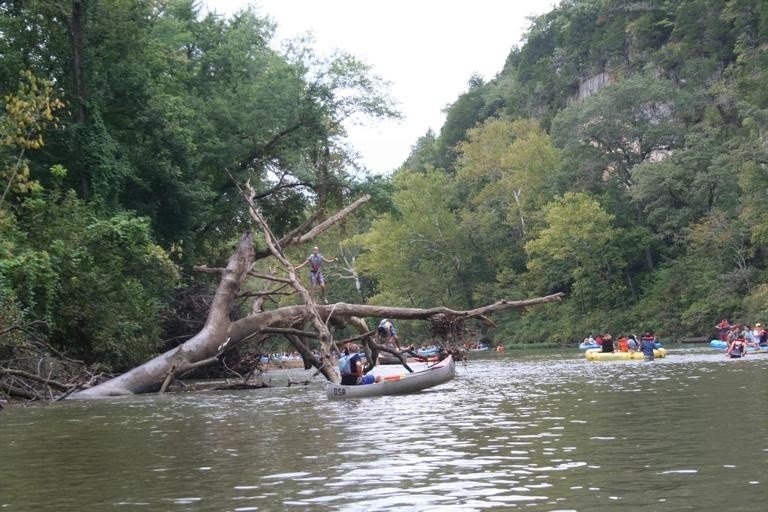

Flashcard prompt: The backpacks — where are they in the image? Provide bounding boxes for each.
[338,353,358,376]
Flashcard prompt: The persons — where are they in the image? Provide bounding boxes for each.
[258,342,484,361]
[338,343,380,386]
[293,246,338,304]
[375,319,400,350]
[578,318,767,358]
[496,343,504,352]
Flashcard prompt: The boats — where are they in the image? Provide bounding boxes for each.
[325,354,458,400]
[727,350,745,358]
[710,340,768,353]
[584,347,665,360]
[257,343,489,359]
[576,342,601,349]
